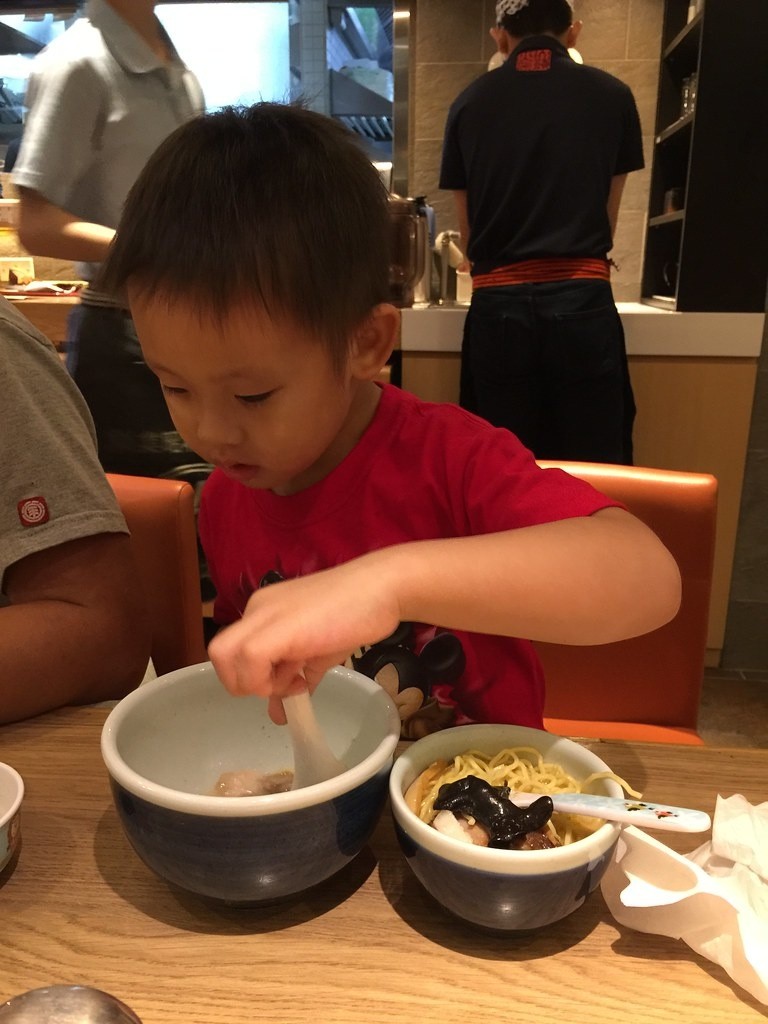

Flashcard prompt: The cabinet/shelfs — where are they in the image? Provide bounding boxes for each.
[641,0,768,312]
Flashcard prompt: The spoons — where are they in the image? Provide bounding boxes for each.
[271,659,350,792]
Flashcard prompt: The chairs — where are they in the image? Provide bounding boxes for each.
[534,456,719,747]
[104,471,208,677]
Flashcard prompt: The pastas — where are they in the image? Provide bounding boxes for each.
[416,744,643,846]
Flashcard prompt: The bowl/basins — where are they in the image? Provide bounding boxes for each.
[0,760,24,876]
[389,723,627,932]
[100,657,401,902]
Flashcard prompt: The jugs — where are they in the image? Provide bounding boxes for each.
[387,199,433,307]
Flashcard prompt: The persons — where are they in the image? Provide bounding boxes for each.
[0,294,150,723]
[438,1,646,465]
[11,0,206,282]
[105,98,682,740]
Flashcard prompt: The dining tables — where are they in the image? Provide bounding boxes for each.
[0,700,768,1024]
